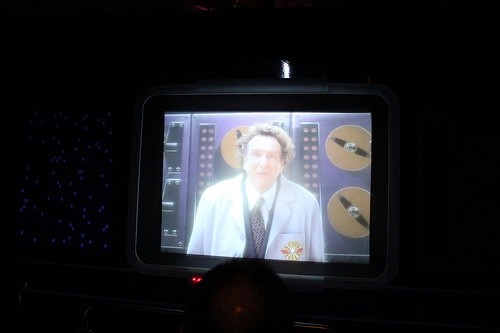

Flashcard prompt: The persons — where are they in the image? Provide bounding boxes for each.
[186,257,295,332]
[188,121,327,262]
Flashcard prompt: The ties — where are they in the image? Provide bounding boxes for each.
[249,196,265,257]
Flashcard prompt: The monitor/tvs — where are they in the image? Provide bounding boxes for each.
[126,84,400,291]
